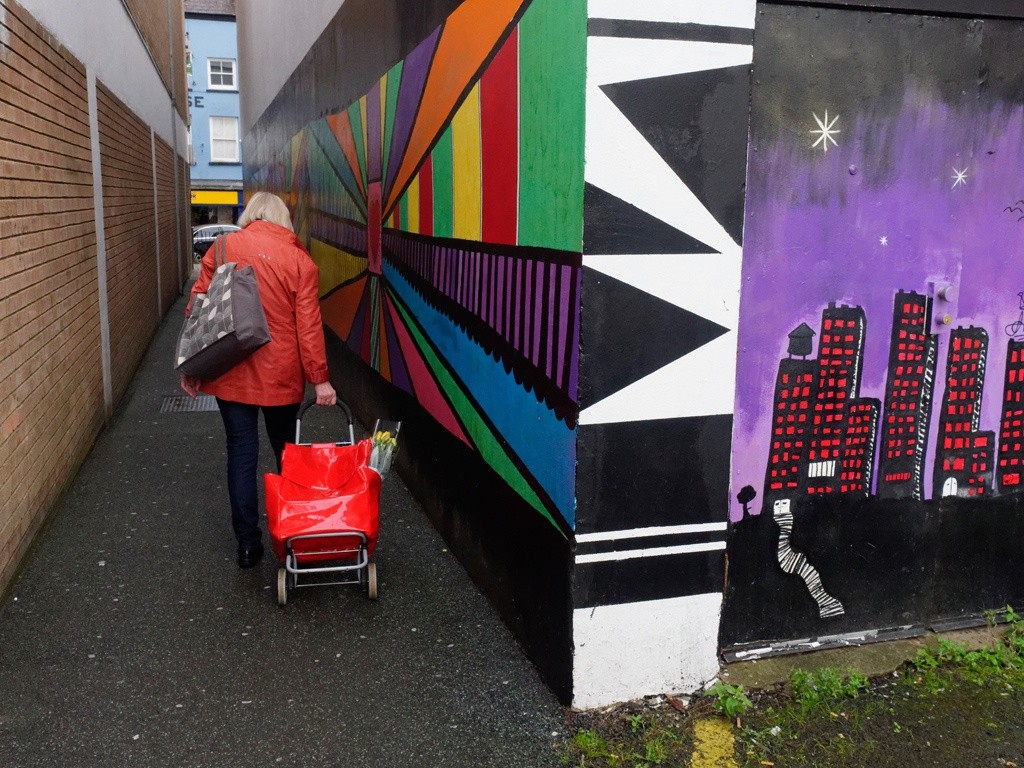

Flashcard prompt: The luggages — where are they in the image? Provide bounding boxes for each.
[264,396,381,605]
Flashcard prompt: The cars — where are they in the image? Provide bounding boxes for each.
[192,224,244,264]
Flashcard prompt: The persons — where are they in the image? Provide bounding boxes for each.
[181,191,337,567]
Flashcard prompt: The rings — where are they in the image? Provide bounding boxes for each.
[326,400,331,402]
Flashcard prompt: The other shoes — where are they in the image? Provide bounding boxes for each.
[239,539,265,568]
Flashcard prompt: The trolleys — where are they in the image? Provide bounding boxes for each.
[277,395,378,606]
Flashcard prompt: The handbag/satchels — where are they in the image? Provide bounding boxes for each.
[173,262,272,382]
